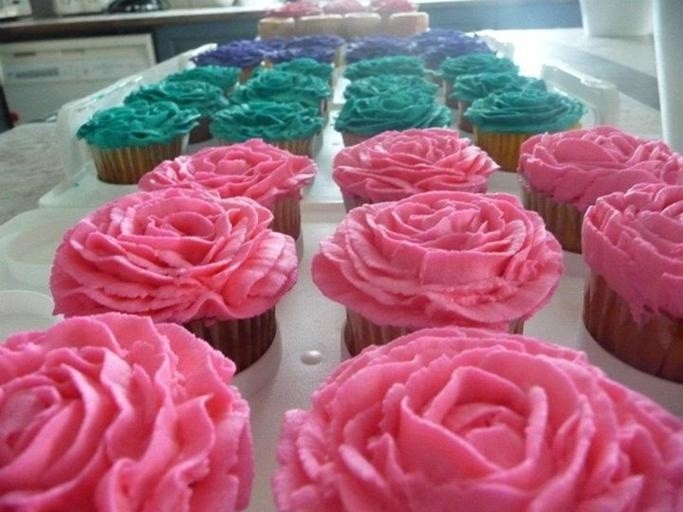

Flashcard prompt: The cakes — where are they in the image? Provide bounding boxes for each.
[332,127,501,215]
[0,311,253,508]
[517,125,683,253]
[50,187,298,375]
[333,95,451,148]
[463,88,587,173]
[312,189,565,356]
[270,326,683,507]
[74,101,201,185]
[454,73,546,132]
[136,138,317,239]
[210,98,325,164]
[256,0,429,41]
[124,81,228,143]
[440,53,518,106]
[162,38,440,92]
[580,180,683,383]
[428,30,493,68]
